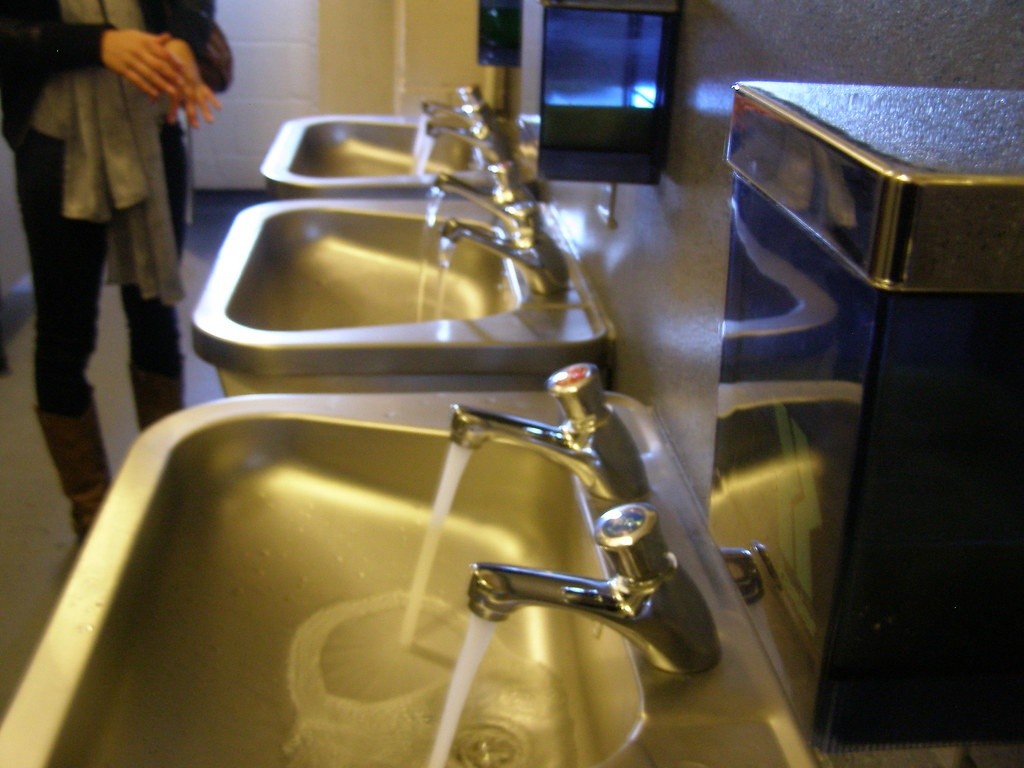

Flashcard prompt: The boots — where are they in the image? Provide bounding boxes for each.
[34,383,111,543]
[129,354,184,432]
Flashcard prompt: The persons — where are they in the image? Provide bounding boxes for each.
[0,0,224,543]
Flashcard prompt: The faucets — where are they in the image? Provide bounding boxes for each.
[420,81,568,295]
[449,361,651,503]
[467,502,722,674]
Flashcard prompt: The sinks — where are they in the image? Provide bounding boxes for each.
[711,380,864,753]
[0,386,815,768]
[190,197,617,398]
[723,213,841,380]
[259,112,485,199]
